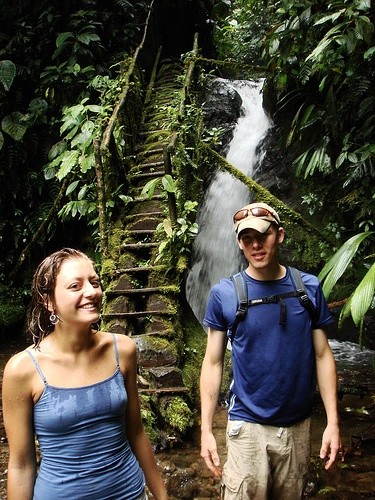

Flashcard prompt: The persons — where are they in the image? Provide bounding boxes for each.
[199,203,344,500]
[2,249,170,500]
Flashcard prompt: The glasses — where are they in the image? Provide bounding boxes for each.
[231,207,280,224]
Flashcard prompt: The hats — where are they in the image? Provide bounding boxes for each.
[233,202,280,240]
